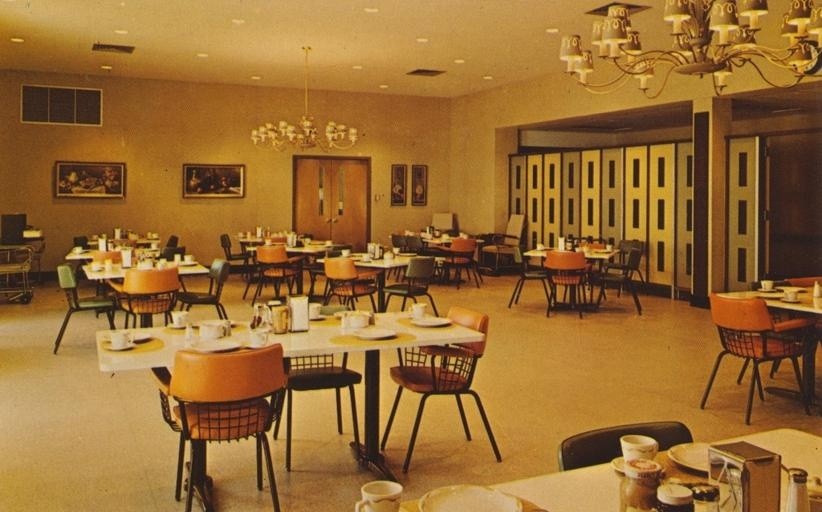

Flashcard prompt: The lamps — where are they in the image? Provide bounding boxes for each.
[556,1,821,100]
[246,47,360,153]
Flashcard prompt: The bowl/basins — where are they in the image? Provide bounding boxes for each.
[419,482,524,512]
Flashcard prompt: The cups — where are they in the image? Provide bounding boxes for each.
[91,262,99,271]
[351,479,403,512]
[392,247,401,256]
[362,253,371,262]
[249,327,269,349]
[341,249,351,258]
[784,289,798,299]
[198,320,228,342]
[92,227,161,255]
[170,310,189,325]
[109,331,134,347]
[173,254,181,264]
[308,302,322,316]
[237,227,298,250]
[184,254,194,263]
[620,432,660,468]
[406,303,428,318]
[106,259,112,271]
[761,280,775,289]
[156,261,166,271]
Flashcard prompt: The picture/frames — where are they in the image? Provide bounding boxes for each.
[181,163,246,199]
[53,160,126,199]
[389,164,407,208]
[411,164,428,208]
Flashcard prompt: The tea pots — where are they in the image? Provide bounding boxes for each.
[138,260,154,271]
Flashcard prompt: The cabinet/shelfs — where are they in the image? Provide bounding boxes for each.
[15,237,49,287]
[0,244,36,306]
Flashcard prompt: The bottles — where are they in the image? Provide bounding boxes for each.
[692,485,720,512]
[650,484,694,512]
[813,280,822,299]
[617,460,661,512]
[556,234,610,255]
[786,467,811,512]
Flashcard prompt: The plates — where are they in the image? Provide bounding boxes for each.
[190,339,240,352]
[128,332,152,341]
[667,442,710,473]
[103,343,136,350]
[757,289,777,292]
[612,457,662,475]
[355,329,397,341]
[780,298,801,302]
[308,315,327,321]
[168,324,186,328]
[413,317,453,327]
